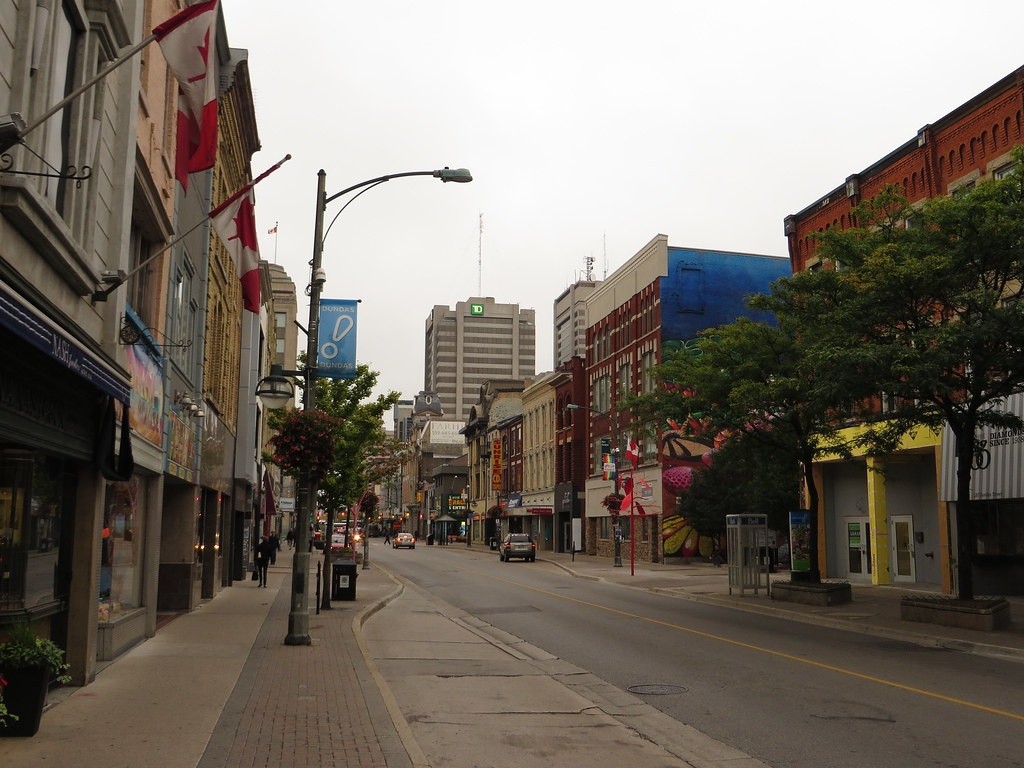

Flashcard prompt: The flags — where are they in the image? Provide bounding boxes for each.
[316,297,359,380]
[152,0,221,194]
[208,158,285,315]
[600,438,611,469]
[625,437,640,470]
[602,453,616,480]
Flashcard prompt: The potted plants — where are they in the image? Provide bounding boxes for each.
[0,616,72,737]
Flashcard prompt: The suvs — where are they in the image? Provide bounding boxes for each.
[501,533,536,564]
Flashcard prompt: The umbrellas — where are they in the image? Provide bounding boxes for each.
[435,515,457,545]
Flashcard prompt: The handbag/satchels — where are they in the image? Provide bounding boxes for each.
[252,566,258,581]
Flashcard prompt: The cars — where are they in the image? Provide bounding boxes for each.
[327,533,351,552]
[392,532,415,549]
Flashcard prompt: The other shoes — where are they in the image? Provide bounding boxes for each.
[258,583,262,587]
[263,585,267,588]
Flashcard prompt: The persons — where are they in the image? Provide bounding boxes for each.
[293,527,297,547]
[269,530,280,565]
[403,529,417,541]
[256,536,273,588]
[384,529,391,545]
[274,531,282,551]
[286,528,293,550]
[308,525,315,552]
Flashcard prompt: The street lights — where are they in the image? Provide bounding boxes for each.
[566,403,623,566]
[283,164,474,647]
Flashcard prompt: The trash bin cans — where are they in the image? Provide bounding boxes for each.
[490,537,497,550]
[426,534,434,545]
[331,558,359,601]
[746,546,779,573]
